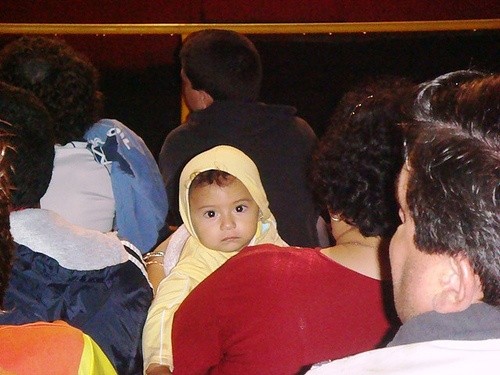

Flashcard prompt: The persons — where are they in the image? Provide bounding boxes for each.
[159,27,330,247]
[172,75,418,375]
[142,145,290,375]
[1,42,115,234]
[22,36,169,257]
[0,188,117,375]
[300,70,500,375]
[0,83,155,375]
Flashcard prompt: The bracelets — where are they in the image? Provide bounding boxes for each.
[143,252,164,260]
[146,261,164,267]
